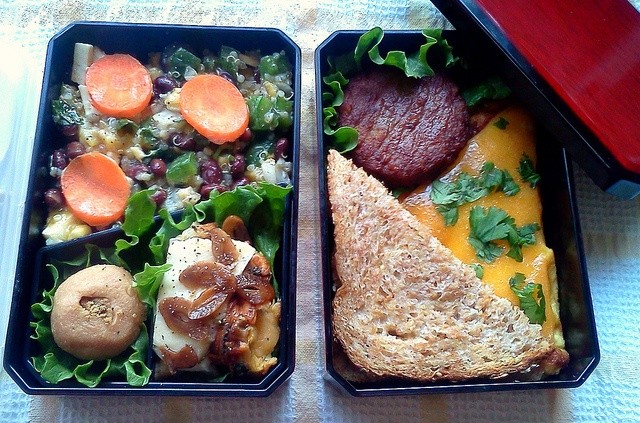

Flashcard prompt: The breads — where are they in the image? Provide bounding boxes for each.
[324,147,571,383]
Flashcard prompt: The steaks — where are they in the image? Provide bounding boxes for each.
[340,61,468,181]
